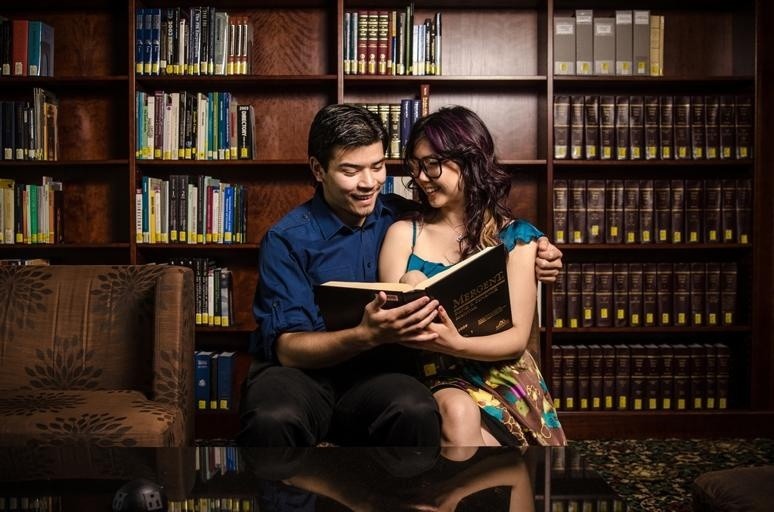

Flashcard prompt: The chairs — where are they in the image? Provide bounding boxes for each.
[2,262,195,510]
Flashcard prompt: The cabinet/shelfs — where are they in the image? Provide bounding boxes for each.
[1,1,772,441]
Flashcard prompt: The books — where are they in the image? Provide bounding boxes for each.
[135,91,258,161]
[135,174,246,245]
[0,175,65,246]
[193,350,235,408]
[0,87,60,161]
[0,427,259,511]
[0,16,55,77]
[135,1,252,76]
[0,258,50,267]
[145,257,235,327]
[551,262,737,328]
[313,243,513,380]
[380,176,419,202]
[356,85,430,160]
[552,94,753,160]
[553,10,664,77]
[551,179,750,246]
[343,1,442,76]
[551,343,732,408]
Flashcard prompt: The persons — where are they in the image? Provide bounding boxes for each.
[376,102,569,460]
[235,101,565,451]
[246,443,384,511]
[383,440,540,512]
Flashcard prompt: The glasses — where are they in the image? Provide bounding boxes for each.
[404,152,456,180]
